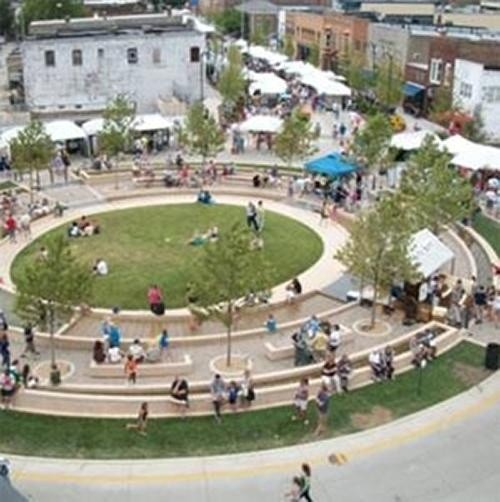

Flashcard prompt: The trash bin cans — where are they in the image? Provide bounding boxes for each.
[486,343,499,369]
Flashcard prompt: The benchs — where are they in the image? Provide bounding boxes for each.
[262,324,355,362]
[88,352,193,378]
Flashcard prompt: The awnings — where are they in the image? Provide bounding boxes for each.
[398,80,424,97]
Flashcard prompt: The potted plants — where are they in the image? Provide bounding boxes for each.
[11,228,99,385]
[328,186,423,351]
[185,217,276,382]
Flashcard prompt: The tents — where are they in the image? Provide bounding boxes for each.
[1,114,174,157]
[240,114,284,133]
[392,130,500,173]
[240,44,351,97]
[403,229,458,288]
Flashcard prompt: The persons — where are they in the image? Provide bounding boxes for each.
[231,54,317,117]
[286,477,301,502]
[1,275,395,437]
[390,260,500,371]
[1,149,113,277]
[328,94,361,149]
[300,463,313,502]
[457,165,500,219]
[129,128,364,252]
